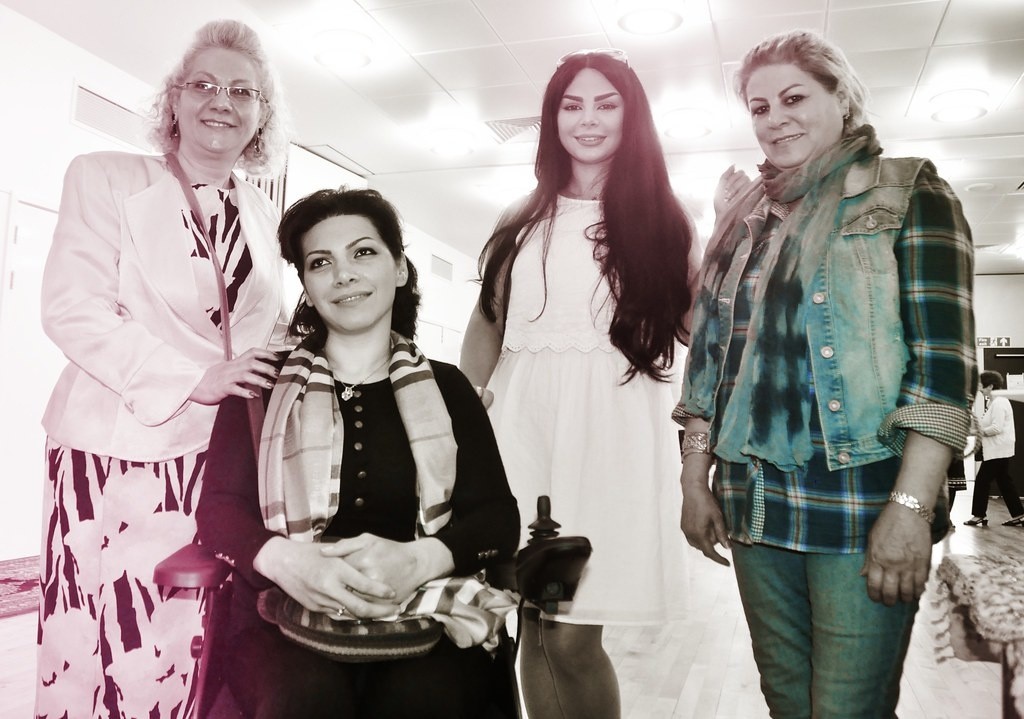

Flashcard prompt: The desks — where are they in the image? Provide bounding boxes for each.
[931,554,1024,719]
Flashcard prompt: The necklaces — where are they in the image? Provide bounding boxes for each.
[331,356,391,402]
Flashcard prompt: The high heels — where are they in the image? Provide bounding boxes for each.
[964,516,988,526]
[1002,516,1024,526]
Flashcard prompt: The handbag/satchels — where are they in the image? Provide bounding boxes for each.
[276,596,443,663]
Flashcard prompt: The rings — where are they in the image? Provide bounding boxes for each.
[337,606,345,616]
[476,386,483,390]
[347,612,350,617]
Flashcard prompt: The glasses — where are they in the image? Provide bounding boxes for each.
[557,47,630,69]
[173,80,269,105]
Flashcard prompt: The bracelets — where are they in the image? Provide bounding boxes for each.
[889,490,935,524]
[681,432,711,463]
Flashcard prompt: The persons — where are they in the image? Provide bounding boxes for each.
[458,53,775,718]
[963,372,1024,529]
[34,19,303,719]
[672,29,976,718]
[194,188,525,719]
